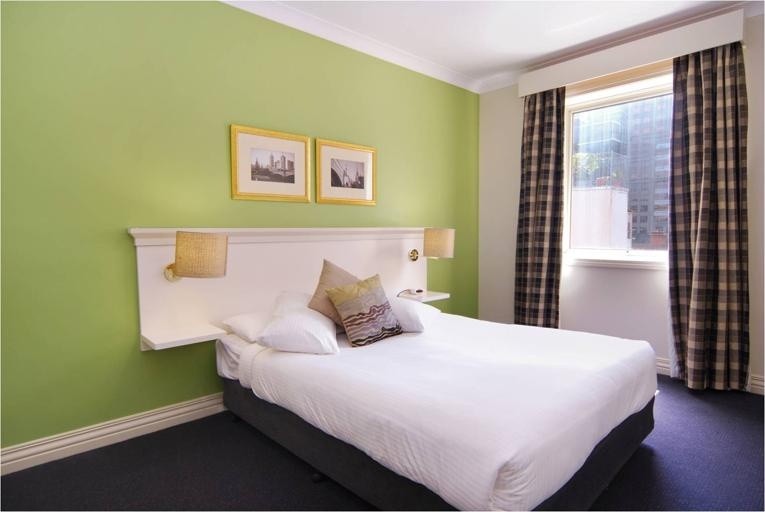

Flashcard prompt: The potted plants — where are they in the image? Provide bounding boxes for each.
[572,152,624,188]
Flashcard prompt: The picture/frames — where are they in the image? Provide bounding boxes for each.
[316,136,378,208]
[230,123,311,203]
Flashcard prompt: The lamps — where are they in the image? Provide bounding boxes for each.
[408,227,455,263]
[163,231,229,283]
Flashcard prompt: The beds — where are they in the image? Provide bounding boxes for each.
[124,227,660,511]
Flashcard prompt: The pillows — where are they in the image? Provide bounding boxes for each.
[388,298,442,332]
[259,293,340,354]
[221,311,267,344]
[324,272,403,347]
[308,258,363,329]
